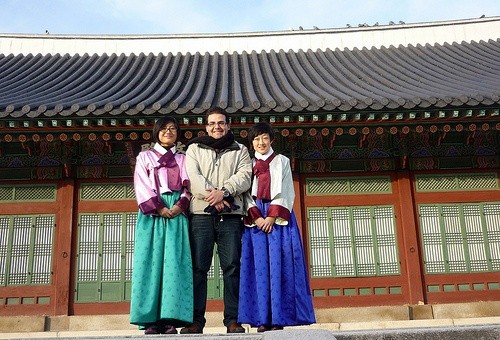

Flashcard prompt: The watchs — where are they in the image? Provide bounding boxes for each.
[222,187,229,197]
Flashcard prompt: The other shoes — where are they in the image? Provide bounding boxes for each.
[271,326,283,330]
[257,325,270,332]
[161,325,177,334]
[145,326,160,334]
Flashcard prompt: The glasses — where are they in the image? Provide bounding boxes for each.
[161,126,177,133]
[207,122,227,127]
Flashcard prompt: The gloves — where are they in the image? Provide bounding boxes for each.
[204,196,240,216]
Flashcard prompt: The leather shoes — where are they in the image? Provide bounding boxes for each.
[180,325,203,333]
[227,322,245,333]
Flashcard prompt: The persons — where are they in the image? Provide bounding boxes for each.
[129,116,194,334]
[180,106,252,334]
[236,121,316,332]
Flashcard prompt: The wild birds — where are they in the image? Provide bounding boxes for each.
[345,22,351,28]
[373,22,379,27]
[44,30,48,35]
[387,20,395,26]
[358,22,369,28]
[398,20,405,24]
[313,25,320,30]
[478,13,486,19]
[297,25,304,30]
